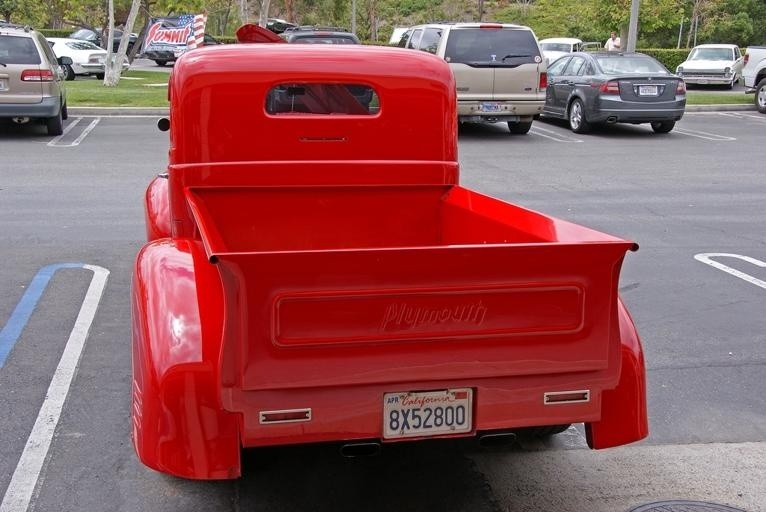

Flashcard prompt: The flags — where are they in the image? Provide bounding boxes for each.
[138,13,209,61]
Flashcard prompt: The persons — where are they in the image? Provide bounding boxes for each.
[604,31,620,51]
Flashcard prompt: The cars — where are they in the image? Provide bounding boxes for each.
[542,50,688,139]
[675,42,745,92]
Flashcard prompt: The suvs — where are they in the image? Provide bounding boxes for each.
[0,22,68,139]
[394,19,551,138]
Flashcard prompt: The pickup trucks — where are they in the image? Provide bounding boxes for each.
[125,42,654,485]
[742,45,766,114]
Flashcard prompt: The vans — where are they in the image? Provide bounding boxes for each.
[537,36,586,65]
